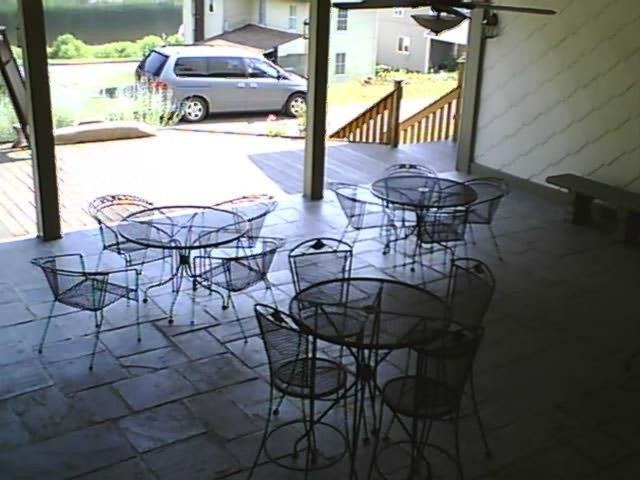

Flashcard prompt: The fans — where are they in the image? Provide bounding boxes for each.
[333,0,558,15]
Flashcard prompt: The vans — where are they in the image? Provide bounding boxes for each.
[135,45,308,122]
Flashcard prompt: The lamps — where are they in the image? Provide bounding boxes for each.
[411,7,467,34]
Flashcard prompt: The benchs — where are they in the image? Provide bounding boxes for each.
[545,173,639,243]
